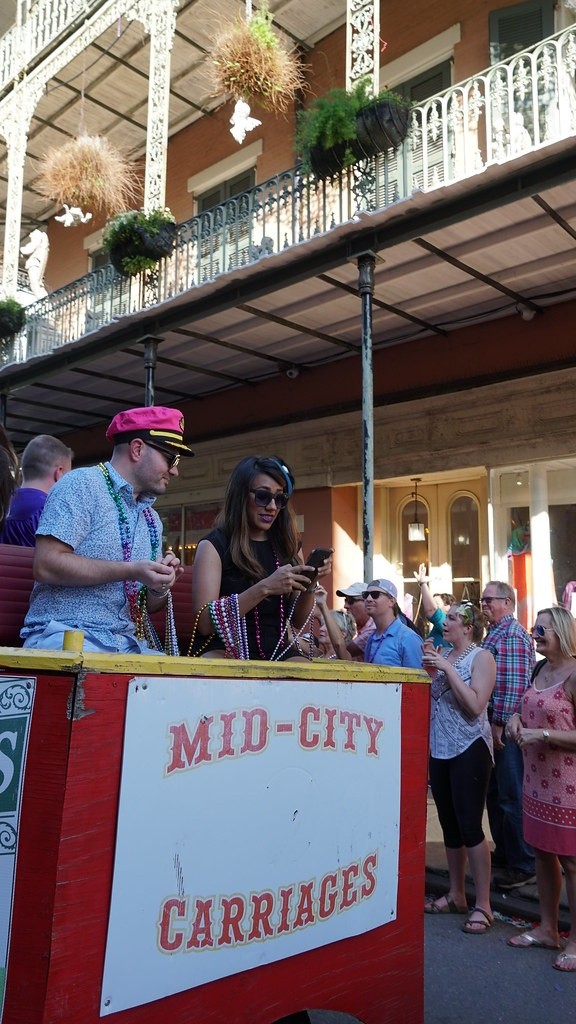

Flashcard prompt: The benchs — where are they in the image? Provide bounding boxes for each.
[0,545,196,654]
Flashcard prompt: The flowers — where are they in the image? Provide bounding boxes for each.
[102,205,177,246]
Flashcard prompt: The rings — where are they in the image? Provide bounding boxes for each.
[162,583,167,589]
[518,740,520,743]
[432,661,433,664]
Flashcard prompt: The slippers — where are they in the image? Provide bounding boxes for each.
[552,952,576,971]
[506,932,560,949]
[424,895,468,914]
[464,908,493,933]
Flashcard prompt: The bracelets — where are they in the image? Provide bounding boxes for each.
[543,729,549,742]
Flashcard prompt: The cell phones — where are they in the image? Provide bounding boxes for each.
[421,643,436,657]
[292,546,332,590]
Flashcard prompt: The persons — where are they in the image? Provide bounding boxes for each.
[481,581,535,888]
[193,456,335,663]
[19,229,55,317]
[506,607,576,970]
[21,406,191,656]
[415,564,454,652]
[420,601,497,932]
[0,424,73,547]
[314,577,424,668]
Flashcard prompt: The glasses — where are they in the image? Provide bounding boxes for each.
[249,488,290,510]
[531,625,554,636]
[344,597,365,605]
[461,599,474,626]
[361,591,393,599]
[481,596,506,604]
[129,439,180,467]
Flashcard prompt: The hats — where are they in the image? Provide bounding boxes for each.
[336,582,368,597]
[368,578,398,598]
[105,406,196,458]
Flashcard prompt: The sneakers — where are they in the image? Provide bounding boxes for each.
[490,851,509,867]
[494,869,538,888]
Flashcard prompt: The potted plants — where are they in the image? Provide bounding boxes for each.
[295,78,409,181]
[0,298,25,339]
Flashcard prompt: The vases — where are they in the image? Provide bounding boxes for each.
[110,221,175,277]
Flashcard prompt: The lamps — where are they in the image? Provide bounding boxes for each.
[407,478,425,542]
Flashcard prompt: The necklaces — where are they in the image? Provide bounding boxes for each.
[435,643,476,679]
[186,557,316,661]
[367,617,398,662]
[97,461,180,656]
[318,654,336,659]
